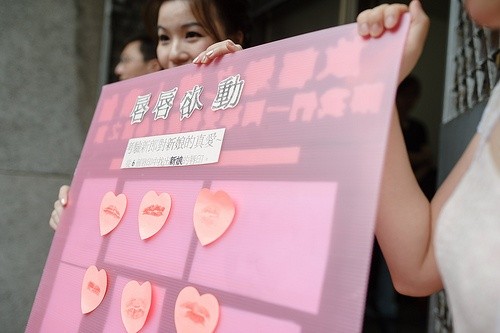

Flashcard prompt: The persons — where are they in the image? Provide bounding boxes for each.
[48,0,245,234]
[353,0,500,333]
[113,37,164,82]
